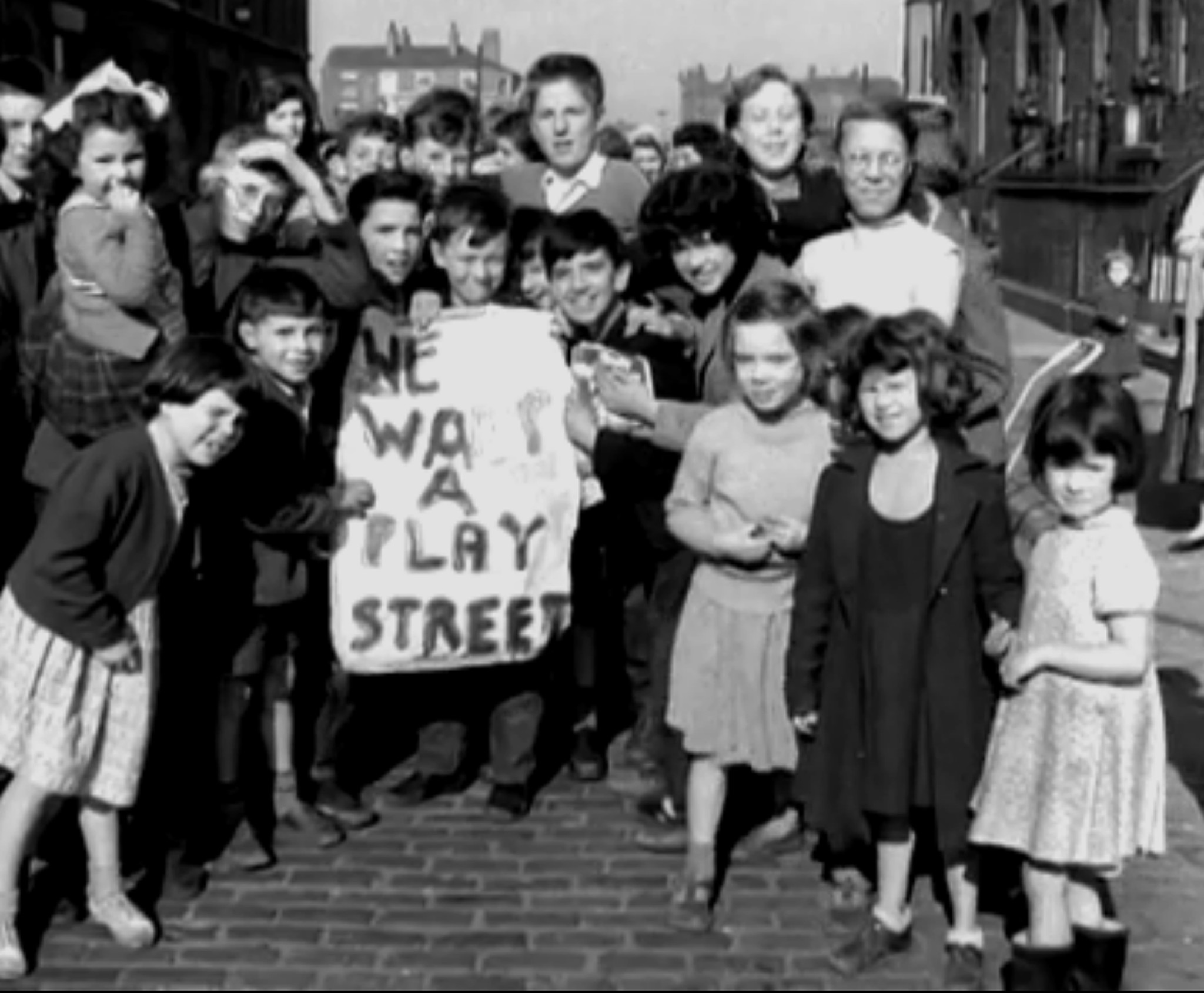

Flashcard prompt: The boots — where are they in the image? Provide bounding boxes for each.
[1072,918,1127,993]
[998,929,1075,992]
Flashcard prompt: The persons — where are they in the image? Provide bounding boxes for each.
[525,206,699,784]
[188,260,376,873]
[0,54,1168,982]
[1169,171,1204,554]
[784,308,1026,993]
[666,282,879,932]
[978,373,1163,972]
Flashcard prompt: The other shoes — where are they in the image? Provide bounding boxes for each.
[942,927,982,992]
[639,822,691,849]
[387,773,458,806]
[0,910,28,981]
[725,827,802,862]
[825,915,913,977]
[158,843,202,900]
[482,777,542,823]
[830,878,870,914]
[88,890,155,946]
[568,728,603,778]
[273,801,339,844]
[670,877,715,930]
[225,819,267,870]
[314,789,374,827]
